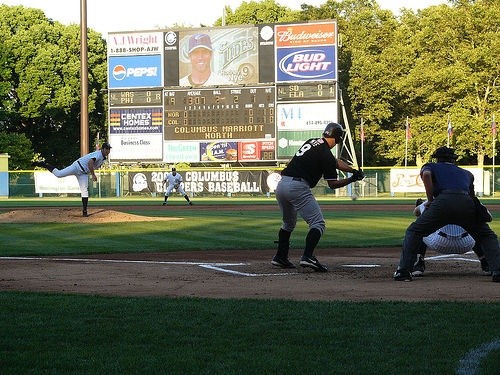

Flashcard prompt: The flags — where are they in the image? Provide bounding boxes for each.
[406,122,411,139]
[360,123,366,141]
[447,117,453,138]
[492,115,497,135]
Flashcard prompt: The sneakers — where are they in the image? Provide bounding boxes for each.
[271,254,296,269]
[299,254,328,272]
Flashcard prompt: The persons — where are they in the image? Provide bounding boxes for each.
[270,123,365,272]
[162,168,193,207]
[393,147,500,283]
[179,34,230,85]
[32,143,112,217]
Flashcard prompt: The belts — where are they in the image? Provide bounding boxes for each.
[281,177,301,181]
[437,231,468,239]
[438,189,469,194]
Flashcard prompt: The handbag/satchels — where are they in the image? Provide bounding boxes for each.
[473,196,492,223]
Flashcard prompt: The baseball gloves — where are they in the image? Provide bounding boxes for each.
[173,183,180,189]
[415,199,426,206]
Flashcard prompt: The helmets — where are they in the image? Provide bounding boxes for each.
[432,146,457,162]
[322,123,344,144]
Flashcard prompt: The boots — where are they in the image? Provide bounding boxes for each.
[184,195,192,206]
[81,197,89,217]
[163,196,169,204]
[31,161,55,173]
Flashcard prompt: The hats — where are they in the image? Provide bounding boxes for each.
[172,168,176,172]
[188,34,213,54]
[102,143,112,148]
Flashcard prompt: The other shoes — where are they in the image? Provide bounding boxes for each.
[411,265,424,277]
[393,269,413,282]
[480,266,492,276]
[492,270,500,282]
